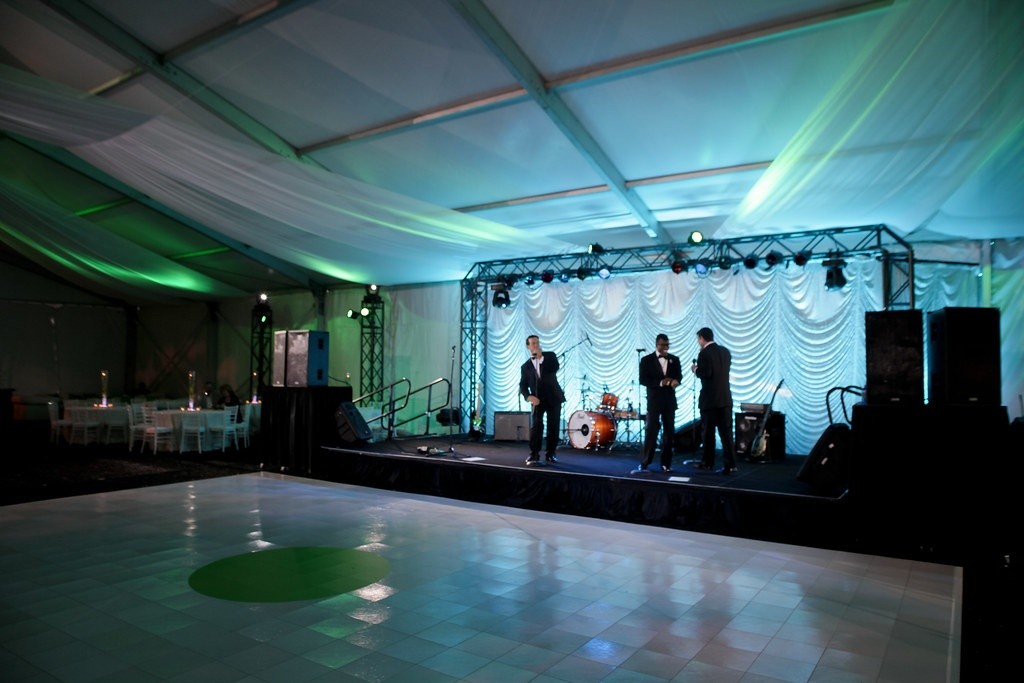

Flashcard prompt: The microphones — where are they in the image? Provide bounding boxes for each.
[692,358,696,365]
[636,349,646,351]
[586,333,592,346]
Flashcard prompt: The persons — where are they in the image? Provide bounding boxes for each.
[202,381,243,432]
[692,328,738,475]
[520,336,566,466]
[638,334,682,472]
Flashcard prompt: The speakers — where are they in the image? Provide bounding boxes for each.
[271,330,330,388]
[865,309,924,405]
[798,423,849,484]
[674,418,701,450]
[735,413,785,456]
[494,411,532,442]
[927,308,1002,407]
[336,401,374,442]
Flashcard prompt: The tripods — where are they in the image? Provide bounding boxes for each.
[608,352,660,450]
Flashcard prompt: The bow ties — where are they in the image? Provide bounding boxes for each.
[658,355,668,359]
[532,356,537,359]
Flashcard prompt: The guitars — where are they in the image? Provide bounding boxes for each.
[746,378,784,458]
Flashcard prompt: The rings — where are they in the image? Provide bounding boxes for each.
[536,401,537,402]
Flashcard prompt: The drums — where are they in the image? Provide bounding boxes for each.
[614,408,636,418]
[567,410,618,449]
[596,392,619,411]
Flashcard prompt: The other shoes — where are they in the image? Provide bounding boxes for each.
[526,456,539,462]
[638,464,646,471]
[662,466,673,471]
[546,455,558,462]
[695,462,712,470]
[724,466,737,472]
